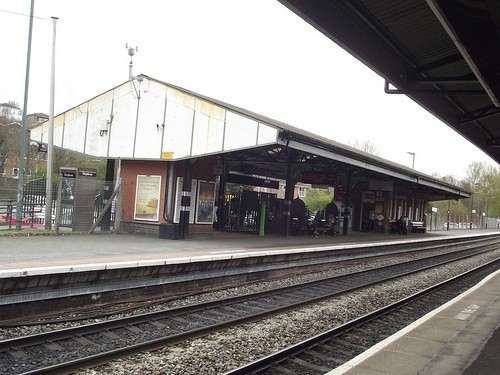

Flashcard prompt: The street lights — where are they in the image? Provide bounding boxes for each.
[406,151,415,170]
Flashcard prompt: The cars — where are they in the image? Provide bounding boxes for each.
[0,195,73,227]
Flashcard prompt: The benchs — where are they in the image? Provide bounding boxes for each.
[409,221,426,234]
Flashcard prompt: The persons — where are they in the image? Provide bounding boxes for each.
[367,208,375,232]
[328,214,335,226]
[401,219,407,235]
